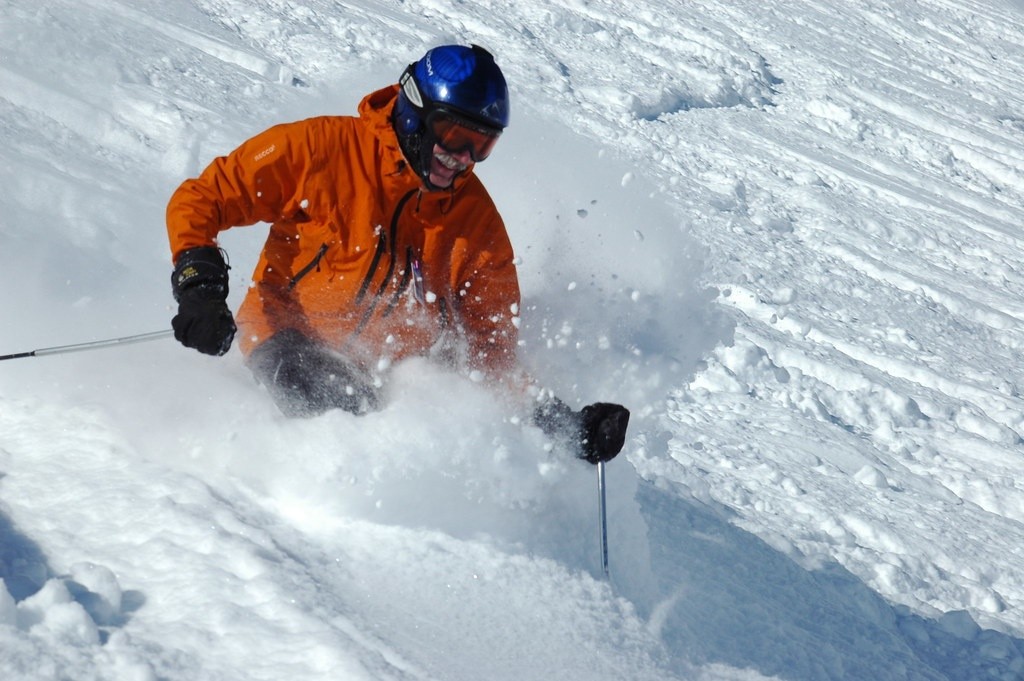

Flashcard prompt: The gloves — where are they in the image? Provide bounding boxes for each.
[171,245,237,357]
[532,397,629,464]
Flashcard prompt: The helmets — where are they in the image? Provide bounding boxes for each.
[395,45,510,135]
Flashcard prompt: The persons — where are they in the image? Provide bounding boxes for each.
[167,44,631,465]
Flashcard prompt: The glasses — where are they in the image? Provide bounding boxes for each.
[425,106,505,162]
